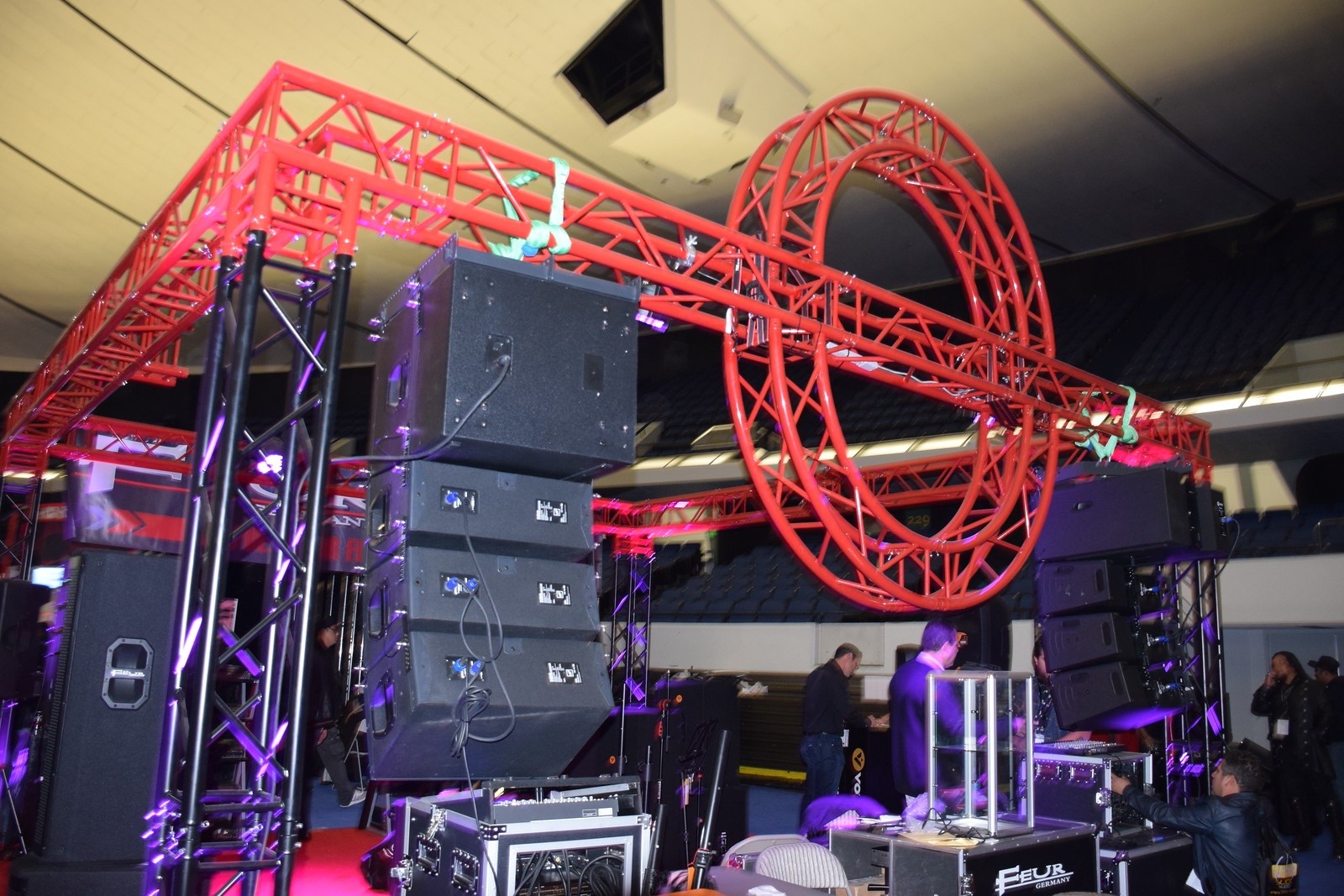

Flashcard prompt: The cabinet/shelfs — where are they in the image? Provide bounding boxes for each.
[926,670,1035,837]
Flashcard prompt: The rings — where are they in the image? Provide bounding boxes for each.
[1268,673,1270,676]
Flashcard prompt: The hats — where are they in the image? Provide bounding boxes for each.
[1308,655,1340,669]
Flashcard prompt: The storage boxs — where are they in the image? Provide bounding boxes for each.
[836,874,885,896]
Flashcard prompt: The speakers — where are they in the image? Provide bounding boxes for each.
[361,245,641,783]
[9,550,181,896]
[1030,466,1233,731]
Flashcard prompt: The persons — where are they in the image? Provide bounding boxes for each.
[888,621,1038,812]
[1250,651,1344,858]
[1108,749,1266,895]
[1000,636,1055,739]
[1307,656,1344,808]
[798,642,875,827]
[307,616,367,808]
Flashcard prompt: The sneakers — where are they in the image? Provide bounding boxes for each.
[339,789,366,808]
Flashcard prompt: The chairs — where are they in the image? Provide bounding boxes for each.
[720,795,886,896]
